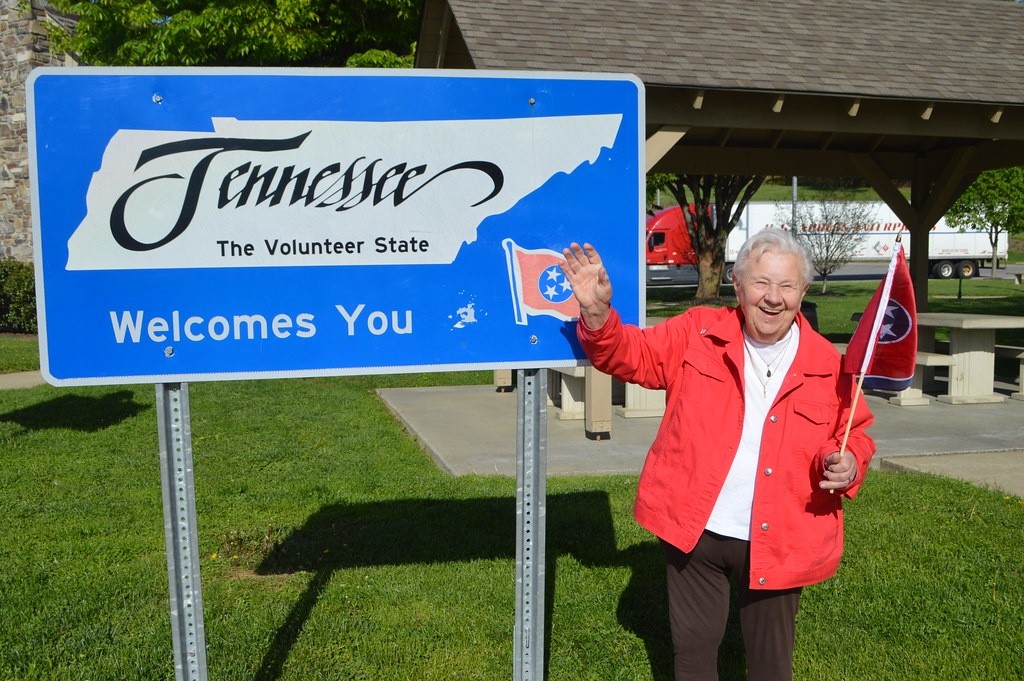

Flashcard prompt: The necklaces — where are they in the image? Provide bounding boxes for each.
[742,325,792,398]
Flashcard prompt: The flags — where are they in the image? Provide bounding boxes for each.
[844,241,918,391]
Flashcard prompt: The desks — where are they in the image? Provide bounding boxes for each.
[850,312,1024,405]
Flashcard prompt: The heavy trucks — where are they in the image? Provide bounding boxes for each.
[647,201,1009,295]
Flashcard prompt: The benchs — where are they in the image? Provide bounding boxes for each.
[493,367,585,420]
[942,340,1024,401]
[833,342,955,405]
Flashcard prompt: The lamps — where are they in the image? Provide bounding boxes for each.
[989,107,1005,123]
[691,91,705,109]
[848,99,862,116]
[920,103,934,120]
[769,96,785,113]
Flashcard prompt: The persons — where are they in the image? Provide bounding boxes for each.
[558,229,876,681]
